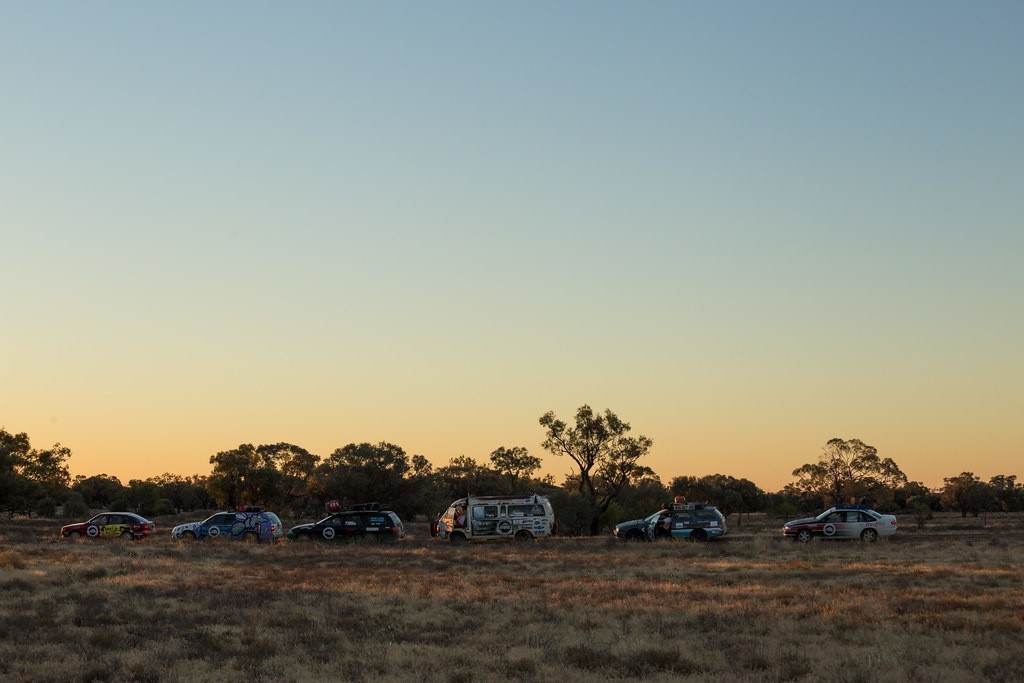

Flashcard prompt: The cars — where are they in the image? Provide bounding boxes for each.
[60,512,156,542]
[170,505,284,546]
[782,495,898,542]
[613,495,728,543]
[287,502,406,544]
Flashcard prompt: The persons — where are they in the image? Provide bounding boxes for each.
[658,514,673,532]
[453,507,465,526]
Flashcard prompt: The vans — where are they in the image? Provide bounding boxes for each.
[430,491,556,547]
